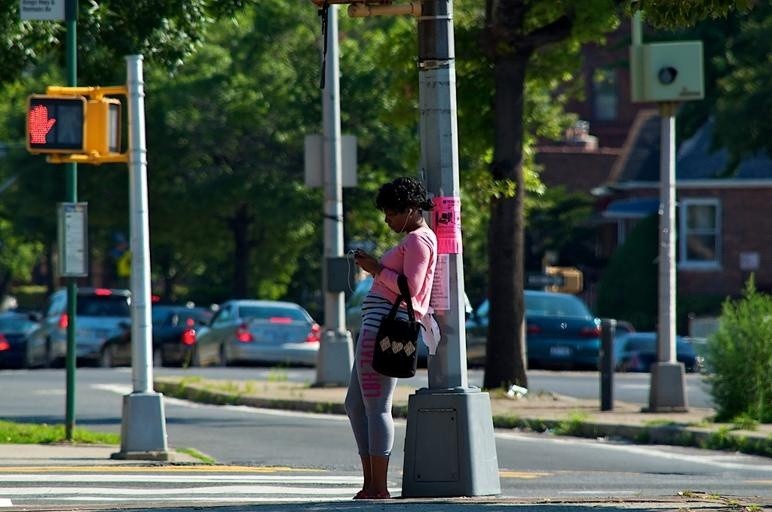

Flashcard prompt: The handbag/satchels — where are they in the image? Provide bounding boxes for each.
[372,273,426,379]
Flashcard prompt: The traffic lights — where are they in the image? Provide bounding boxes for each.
[22,95,87,162]
[86,97,122,155]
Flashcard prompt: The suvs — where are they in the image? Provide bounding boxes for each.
[24,287,134,369]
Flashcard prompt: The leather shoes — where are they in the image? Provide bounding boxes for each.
[357,491,390,499]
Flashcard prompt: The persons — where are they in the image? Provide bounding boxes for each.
[342,177,442,499]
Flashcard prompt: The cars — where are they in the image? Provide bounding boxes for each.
[347,275,471,371]
[98,305,212,368]
[1,312,38,369]
[192,299,324,366]
[612,329,698,373]
[465,290,601,371]
